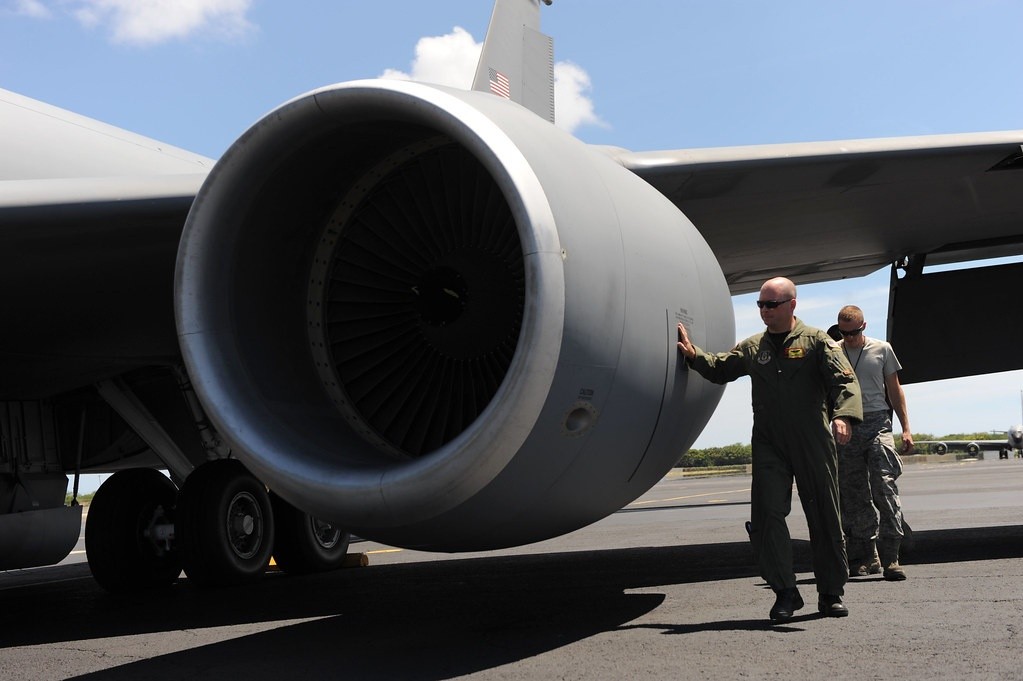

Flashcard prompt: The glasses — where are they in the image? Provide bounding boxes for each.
[757,299,792,309]
[839,322,864,336]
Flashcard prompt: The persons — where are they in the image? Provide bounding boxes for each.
[825,305,912,580]
[678,277,864,622]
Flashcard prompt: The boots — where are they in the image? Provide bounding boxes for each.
[876,537,906,580]
[850,539,881,576]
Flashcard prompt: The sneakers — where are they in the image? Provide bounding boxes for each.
[769,586,804,620]
[818,593,848,616]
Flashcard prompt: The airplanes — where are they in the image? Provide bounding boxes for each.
[914,426,1023,460]
[1,0,1023,610]
